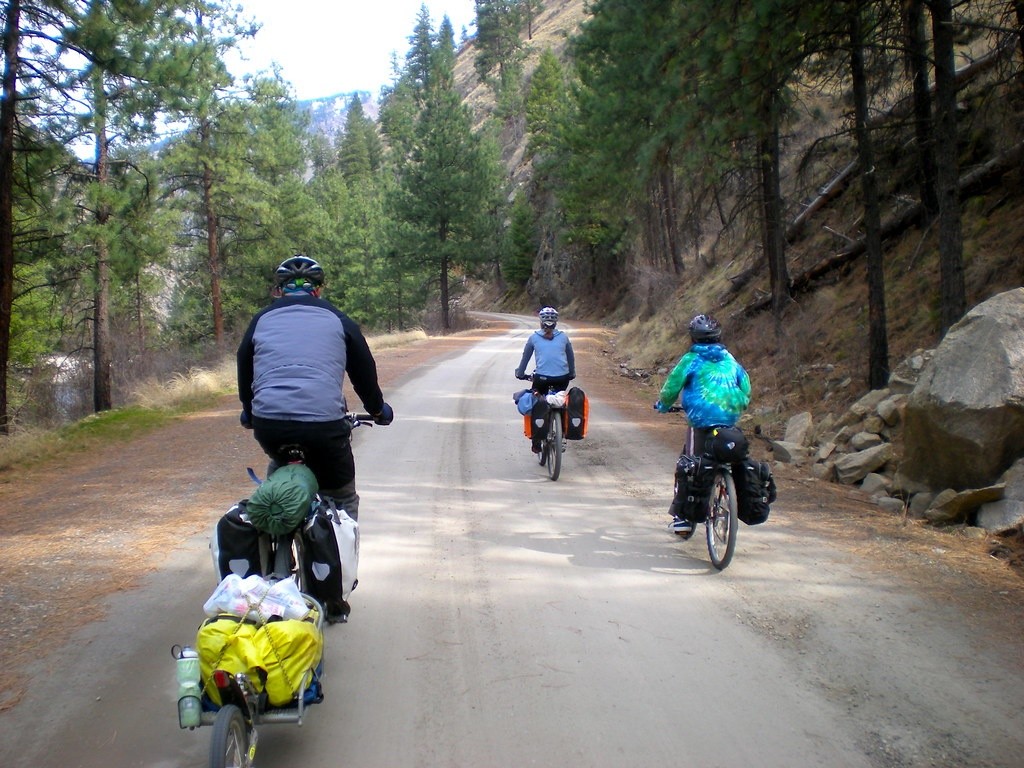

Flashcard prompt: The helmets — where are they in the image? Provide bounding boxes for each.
[275,254,324,289]
[688,315,722,344]
[539,306,558,329]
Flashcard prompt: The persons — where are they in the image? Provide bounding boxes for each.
[237,256,393,618]
[656,314,751,531]
[515,307,576,453]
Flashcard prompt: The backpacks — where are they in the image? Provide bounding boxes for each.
[732,457,776,525]
[675,455,715,522]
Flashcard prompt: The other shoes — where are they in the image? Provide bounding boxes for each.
[561,433,567,453]
[531,438,542,453]
[326,599,350,623]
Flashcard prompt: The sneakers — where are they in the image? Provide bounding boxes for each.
[668,513,692,531]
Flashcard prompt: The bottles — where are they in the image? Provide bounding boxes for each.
[171,643,203,728]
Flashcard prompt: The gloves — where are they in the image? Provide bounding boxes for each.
[515,368,524,378]
[372,403,393,425]
[656,400,668,413]
[568,371,576,379]
[240,411,254,429]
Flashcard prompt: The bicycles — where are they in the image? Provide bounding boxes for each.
[652,404,740,571]
[169,412,389,768]
[514,371,575,482]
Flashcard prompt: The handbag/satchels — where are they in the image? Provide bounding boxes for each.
[291,493,360,602]
[564,387,588,440]
[524,392,549,438]
[202,574,310,625]
[209,498,272,581]
[195,599,323,712]
